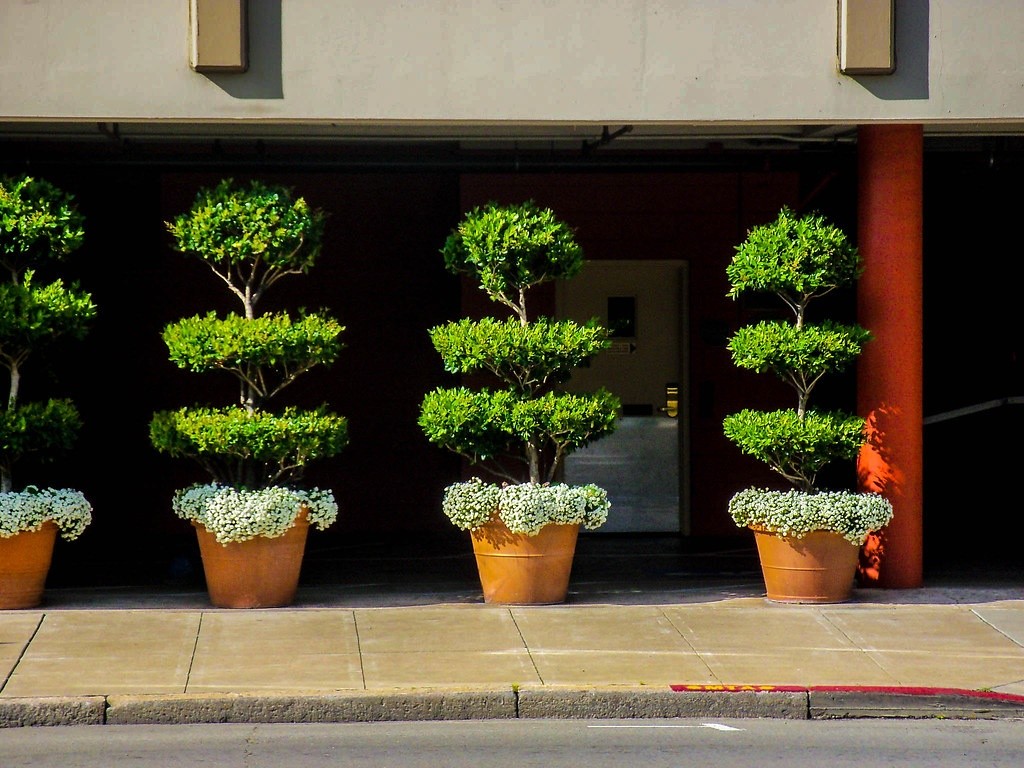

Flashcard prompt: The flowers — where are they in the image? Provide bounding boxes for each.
[417,198,623,536]
[721,203,894,546]
[1,170,98,543]
[147,176,349,546]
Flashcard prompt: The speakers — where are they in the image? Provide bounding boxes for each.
[836,0,897,75]
[189,0,248,72]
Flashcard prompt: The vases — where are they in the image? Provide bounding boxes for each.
[189,500,311,607]
[1,519,56,610]
[468,519,580,607]
[748,523,860,604]
